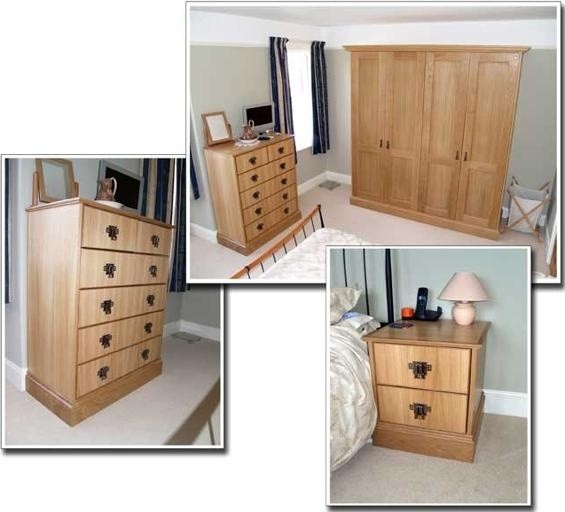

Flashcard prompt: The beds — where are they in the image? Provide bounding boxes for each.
[224,206,371,280]
[330,249,397,475]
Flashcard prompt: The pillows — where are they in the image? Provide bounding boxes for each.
[330,284,361,325]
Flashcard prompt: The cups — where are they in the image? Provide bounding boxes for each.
[402,308,415,319]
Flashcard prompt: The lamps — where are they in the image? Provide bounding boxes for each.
[439,272,487,327]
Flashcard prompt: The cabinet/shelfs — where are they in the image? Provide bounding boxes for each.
[418,43,532,242]
[341,41,426,225]
[25,199,173,430]
[203,130,303,256]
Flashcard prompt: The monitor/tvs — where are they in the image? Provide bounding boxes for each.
[96,160,145,215]
[242,103,275,140]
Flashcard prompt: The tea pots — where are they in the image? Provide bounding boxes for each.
[241,120,257,140]
[95,177,117,201]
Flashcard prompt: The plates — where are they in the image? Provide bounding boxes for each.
[92,200,124,209]
[237,137,259,144]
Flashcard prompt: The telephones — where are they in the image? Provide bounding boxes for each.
[411,287,442,321]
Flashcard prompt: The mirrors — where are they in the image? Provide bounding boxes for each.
[30,157,80,206]
[201,111,233,145]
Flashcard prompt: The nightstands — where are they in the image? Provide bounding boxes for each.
[362,315,493,466]
